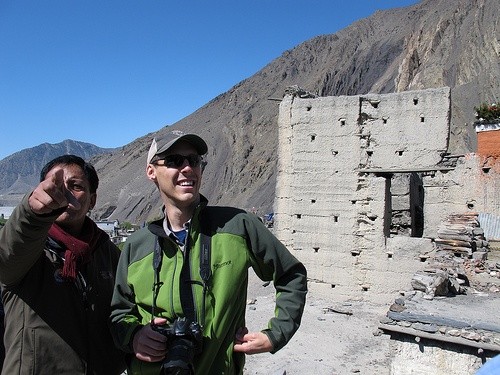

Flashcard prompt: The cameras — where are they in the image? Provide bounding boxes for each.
[151,316,205,375]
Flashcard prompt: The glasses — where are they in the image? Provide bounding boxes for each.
[151,154,204,168]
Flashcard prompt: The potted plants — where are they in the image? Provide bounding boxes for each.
[473,102,500,120]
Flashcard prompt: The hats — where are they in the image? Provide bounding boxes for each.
[146,130,209,167]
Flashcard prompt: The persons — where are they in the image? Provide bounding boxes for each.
[109,130,308,375]
[0,155,126,375]
